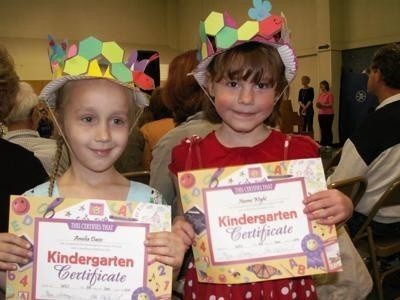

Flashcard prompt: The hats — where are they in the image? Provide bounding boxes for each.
[40,31,154,110]
[192,0,301,83]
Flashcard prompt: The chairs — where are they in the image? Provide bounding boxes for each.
[324,174,368,209]
[119,171,150,185]
[349,179,399,300]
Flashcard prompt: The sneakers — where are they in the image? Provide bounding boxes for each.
[319,145,333,150]
